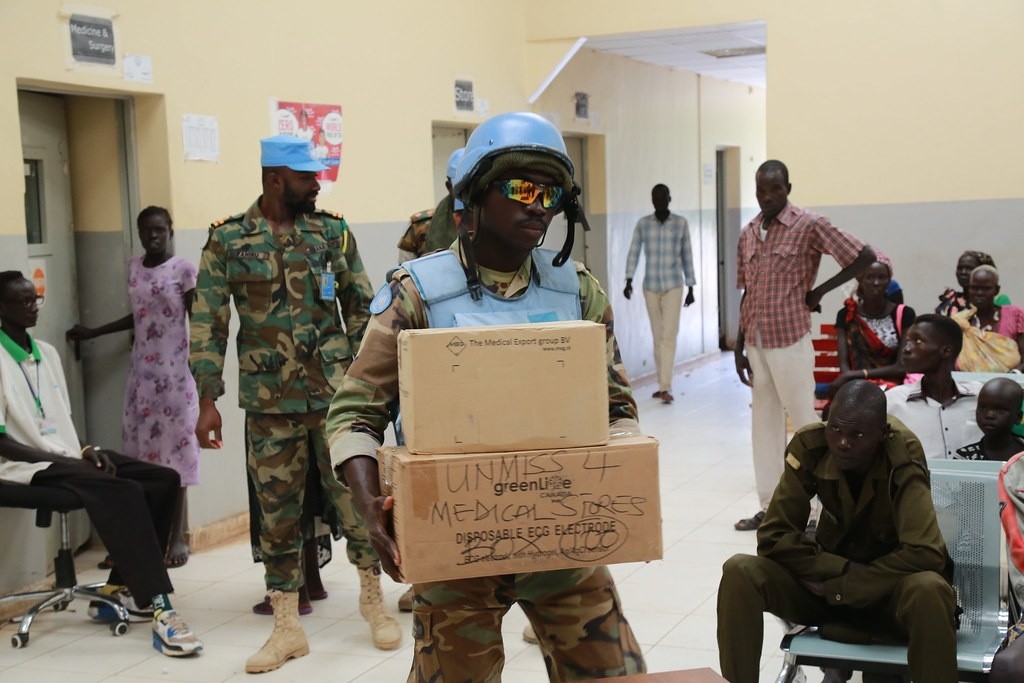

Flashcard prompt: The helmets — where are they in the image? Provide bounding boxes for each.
[455,111,574,200]
[446,148,465,178]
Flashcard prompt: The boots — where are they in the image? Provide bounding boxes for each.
[358,563,401,647]
[245,588,309,673]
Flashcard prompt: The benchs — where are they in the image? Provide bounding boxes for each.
[774,458,1024,683]
[813,324,854,410]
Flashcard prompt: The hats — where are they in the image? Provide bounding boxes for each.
[261,136,330,172]
[469,152,573,215]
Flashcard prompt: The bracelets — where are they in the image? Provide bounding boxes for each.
[81,445,92,455]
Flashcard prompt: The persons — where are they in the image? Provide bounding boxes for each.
[733,159,876,539]
[0,271,204,657]
[717,251,1024,683]
[188,112,647,683]
[65,206,200,570]
[623,185,695,401]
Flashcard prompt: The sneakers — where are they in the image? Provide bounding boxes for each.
[152,611,202,655]
[87,585,154,622]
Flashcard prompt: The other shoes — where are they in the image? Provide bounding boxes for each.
[253,596,311,614]
[805,520,817,541]
[735,511,765,529]
[298,585,327,599]
[653,391,662,398]
[662,391,673,403]
[399,586,413,611]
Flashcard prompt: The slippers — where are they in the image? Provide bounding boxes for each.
[165,533,190,567]
[98,554,114,568]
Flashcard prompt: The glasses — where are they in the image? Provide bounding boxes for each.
[0,295,44,306]
[491,178,564,207]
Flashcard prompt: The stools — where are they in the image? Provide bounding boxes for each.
[0,485,129,649]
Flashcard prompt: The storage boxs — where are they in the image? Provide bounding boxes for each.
[397,320,609,454]
[376,428,664,583]
